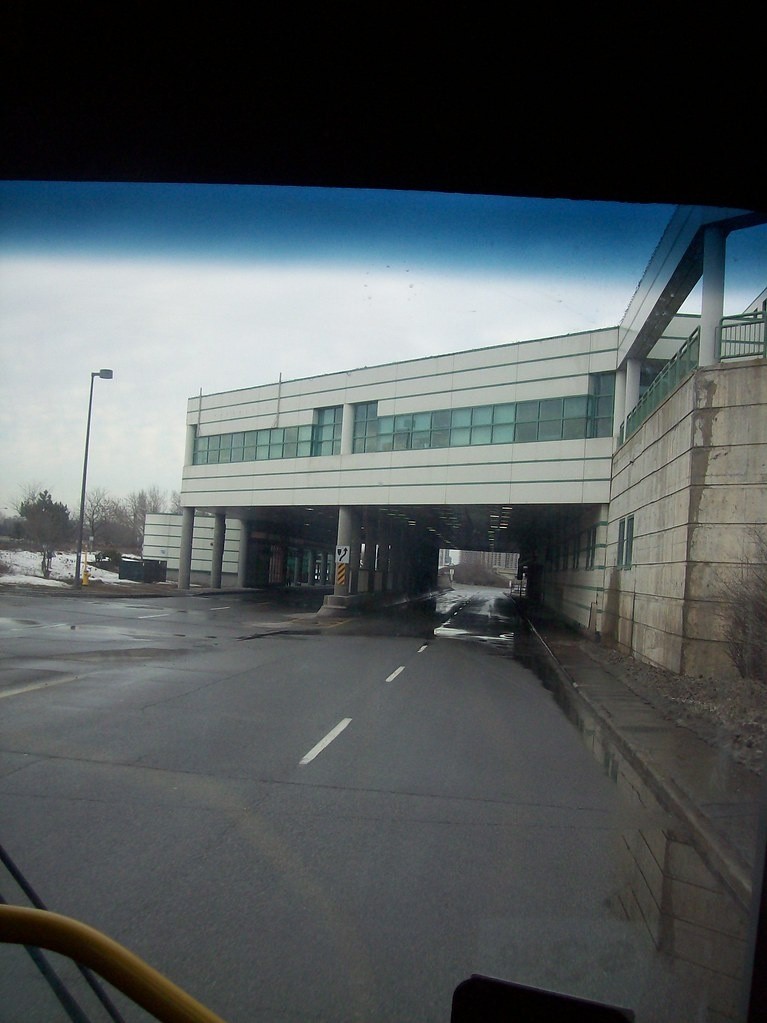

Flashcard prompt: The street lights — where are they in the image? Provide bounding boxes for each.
[75,369,113,579]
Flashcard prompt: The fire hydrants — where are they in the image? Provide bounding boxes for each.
[82,551,91,586]
